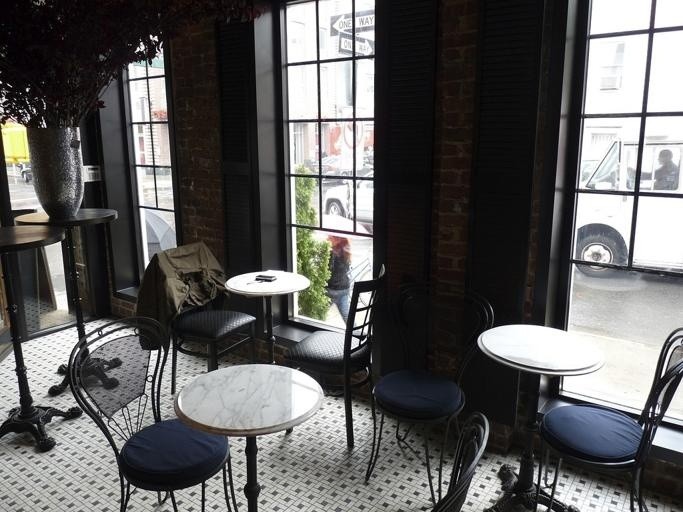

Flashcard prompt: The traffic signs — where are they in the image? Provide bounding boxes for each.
[339,34,375,60]
[330,10,375,34]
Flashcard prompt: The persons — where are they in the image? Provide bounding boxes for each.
[323,232,352,326]
[624,149,678,189]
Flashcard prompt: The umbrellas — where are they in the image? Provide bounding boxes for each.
[312,213,370,241]
[144,207,177,261]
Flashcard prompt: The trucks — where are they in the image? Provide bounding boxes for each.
[574,124,683,277]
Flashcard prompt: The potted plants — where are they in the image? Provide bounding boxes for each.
[0,0,269,218]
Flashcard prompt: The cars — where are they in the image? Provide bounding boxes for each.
[312,153,374,184]
[21,165,33,182]
[324,171,373,220]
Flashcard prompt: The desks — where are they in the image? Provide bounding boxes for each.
[0,226,82,453]
[14,208,118,397]
